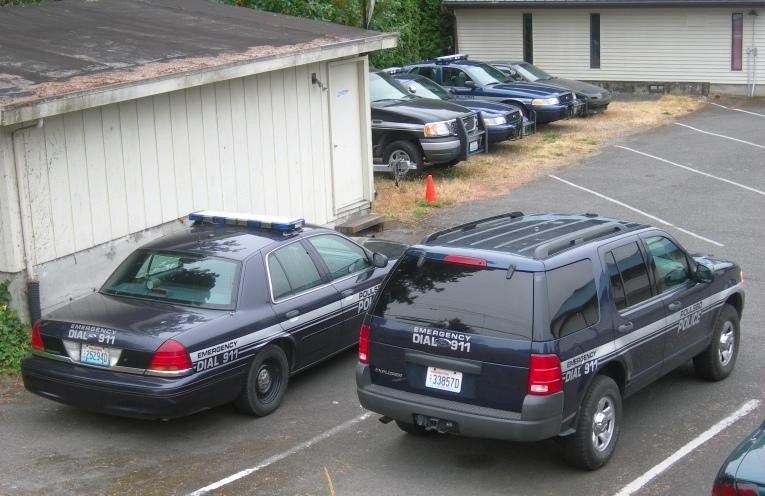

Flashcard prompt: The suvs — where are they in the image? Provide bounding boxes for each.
[351,207,747,475]
[367,65,484,182]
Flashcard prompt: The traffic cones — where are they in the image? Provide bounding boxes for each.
[423,173,438,204]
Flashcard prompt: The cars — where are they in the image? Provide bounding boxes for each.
[17,202,407,427]
[384,72,523,145]
[393,51,585,131]
[706,414,765,495]
[482,61,613,115]
